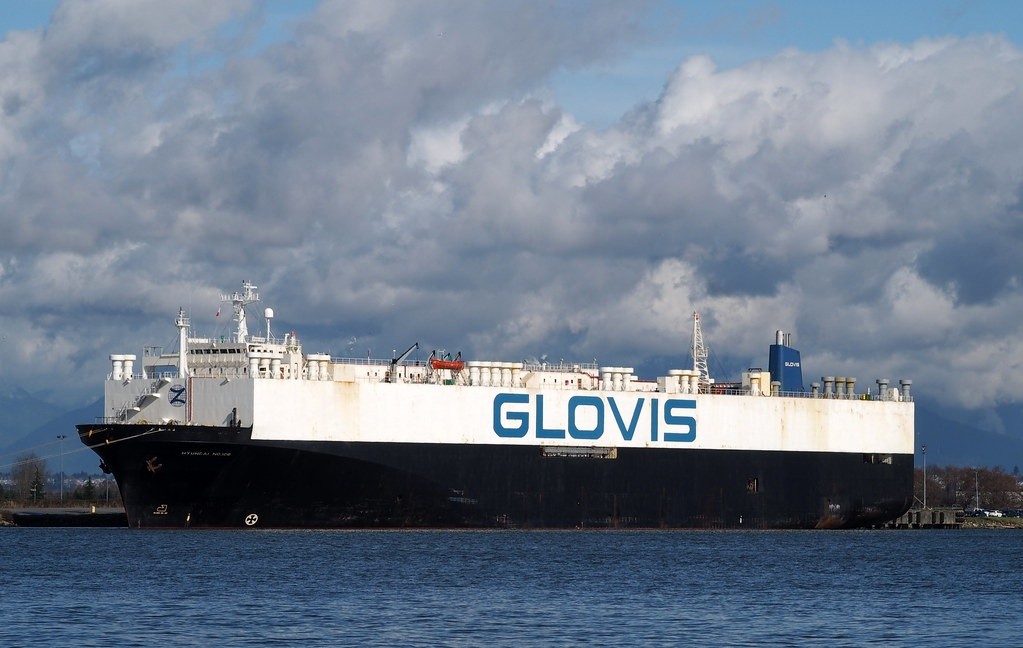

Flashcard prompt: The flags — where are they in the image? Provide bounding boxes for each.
[216,308,220,316]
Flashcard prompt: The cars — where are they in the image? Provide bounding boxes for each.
[966,506,1023,518]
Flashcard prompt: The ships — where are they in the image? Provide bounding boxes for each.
[72,279,920,531]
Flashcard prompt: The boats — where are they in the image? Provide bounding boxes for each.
[432,359,465,372]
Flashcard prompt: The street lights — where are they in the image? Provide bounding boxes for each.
[921,444,927,508]
[57,433,69,504]
[975,468,980,509]
